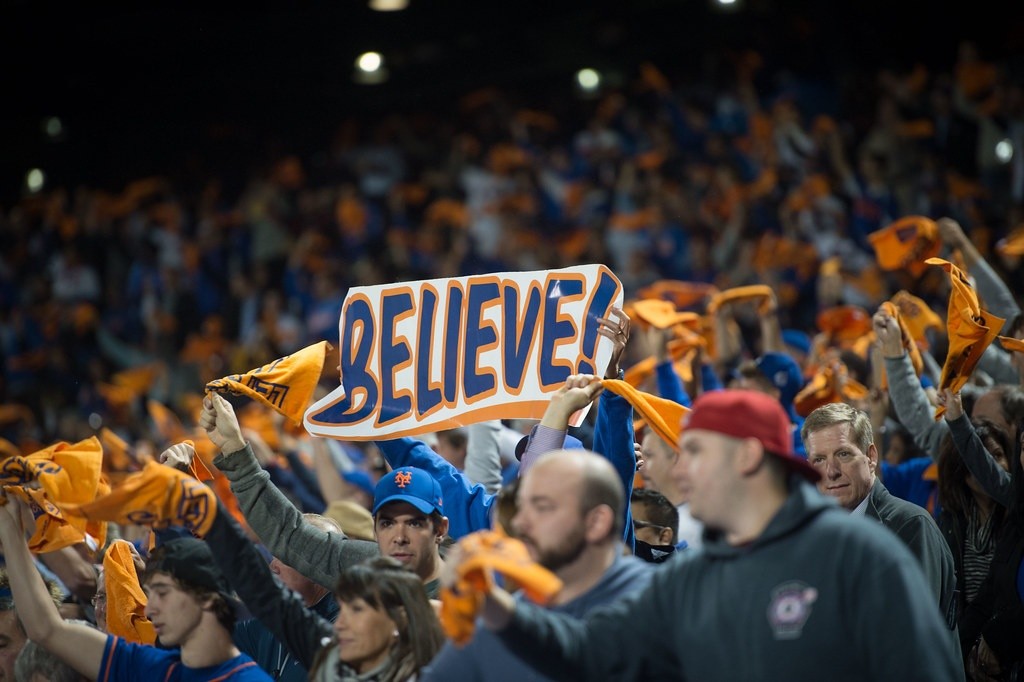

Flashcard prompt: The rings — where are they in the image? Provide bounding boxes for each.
[617,330,623,335]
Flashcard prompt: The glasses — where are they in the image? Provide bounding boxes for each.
[633,518,670,531]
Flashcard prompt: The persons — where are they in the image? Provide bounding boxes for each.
[160,443,447,682]
[802,403,957,633]
[0,485,276,681]
[449,390,964,682]
[0,52,1024,682]
[202,391,448,605]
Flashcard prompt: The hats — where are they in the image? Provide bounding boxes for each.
[754,351,805,395]
[372,467,444,516]
[679,389,823,485]
[149,537,247,616]
